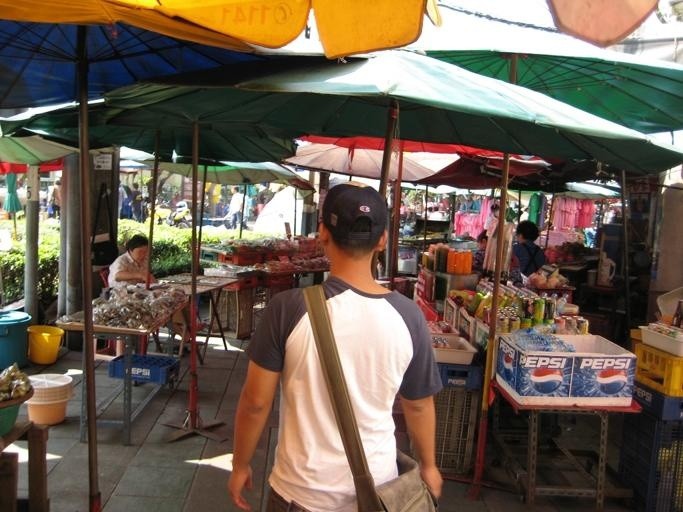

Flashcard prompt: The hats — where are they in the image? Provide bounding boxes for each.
[322,181,387,240]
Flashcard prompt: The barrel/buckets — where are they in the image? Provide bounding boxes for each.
[0,310,32,371]
[27,323,67,365]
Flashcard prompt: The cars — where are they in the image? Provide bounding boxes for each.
[0,177,60,210]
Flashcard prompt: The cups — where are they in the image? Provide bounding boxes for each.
[588,269,598,287]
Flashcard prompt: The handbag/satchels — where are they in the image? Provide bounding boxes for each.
[359,445,439,512]
[91,240,118,266]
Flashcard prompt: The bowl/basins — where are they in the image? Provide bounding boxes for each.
[0,380,34,434]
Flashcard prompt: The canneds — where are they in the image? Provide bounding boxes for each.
[484,297,588,334]
[427,320,450,348]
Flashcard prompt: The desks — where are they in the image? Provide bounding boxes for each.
[55,257,328,447]
[400,293,646,510]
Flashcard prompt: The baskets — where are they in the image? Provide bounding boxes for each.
[209,286,253,339]
[616,404,683,512]
[432,387,480,475]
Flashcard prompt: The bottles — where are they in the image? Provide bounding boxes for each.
[509,327,574,353]
[427,243,474,274]
[672,298,683,329]
[543,291,569,315]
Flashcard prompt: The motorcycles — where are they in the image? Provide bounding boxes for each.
[145,199,191,228]
